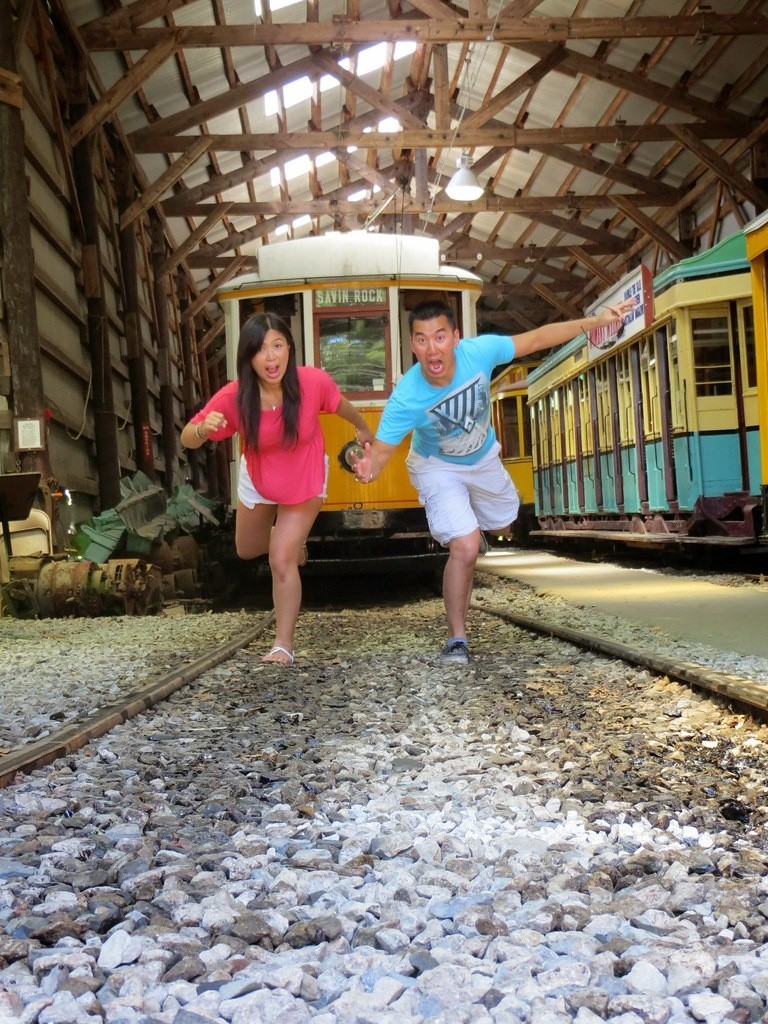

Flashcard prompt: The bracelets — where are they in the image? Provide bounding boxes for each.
[196,421,206,442]
[354,472,374,484]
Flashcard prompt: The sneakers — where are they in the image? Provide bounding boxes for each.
[478,529,488,555]
[441,638,469,664]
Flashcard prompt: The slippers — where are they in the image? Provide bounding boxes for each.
[262,646,296,665]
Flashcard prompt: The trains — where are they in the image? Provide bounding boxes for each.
[491,208,768,562]
[213,229,489,587]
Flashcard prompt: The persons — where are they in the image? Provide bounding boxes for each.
[351,300,637,663]
[181,312,373,666]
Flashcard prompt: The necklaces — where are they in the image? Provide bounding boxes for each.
[271,404,277,413]
